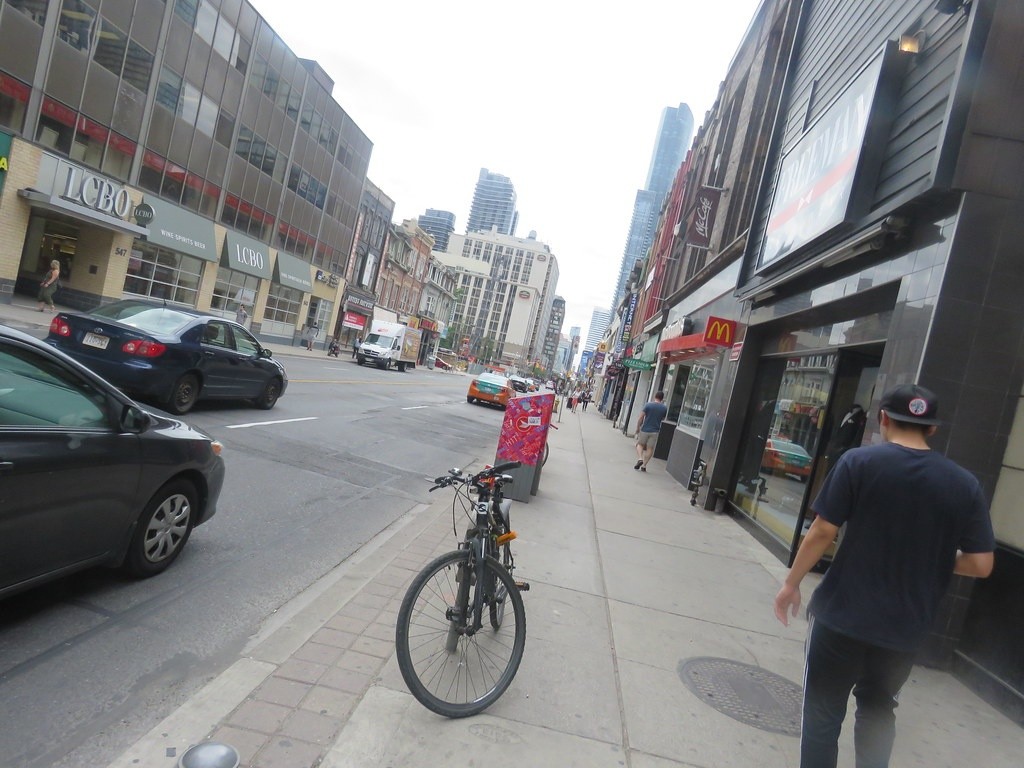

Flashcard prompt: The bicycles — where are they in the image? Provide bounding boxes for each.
[542,422,560,470]
[396,458,532,718]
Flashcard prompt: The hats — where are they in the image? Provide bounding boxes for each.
[878,383,944,427]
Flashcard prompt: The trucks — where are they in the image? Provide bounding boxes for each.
[356,319,421,372]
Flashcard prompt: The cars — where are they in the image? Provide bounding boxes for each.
[508,374,554,395]
[435,355,452,370]
[760,437,813,486]
[466,372,516,408]
[41,298,291,415]
[0,327,226,610]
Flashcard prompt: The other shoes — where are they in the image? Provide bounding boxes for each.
[641,467,646,471]
[634,460,643,469]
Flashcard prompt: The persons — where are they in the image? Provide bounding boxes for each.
[559,381,592,413]
[34,258,60,313]
[306,321,319,351]
[236,303,248,326]
[825,401,866,481]
[771,383,1000,768]
[633,390,669,473]
[351,335,362,359]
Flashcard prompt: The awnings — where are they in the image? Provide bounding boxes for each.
[219,229,271,282]
[270,250,313,296]
[138,195,219,264]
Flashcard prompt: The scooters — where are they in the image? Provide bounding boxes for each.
[326,334,341,357]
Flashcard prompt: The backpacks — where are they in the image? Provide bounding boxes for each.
[57,276,62,289]
